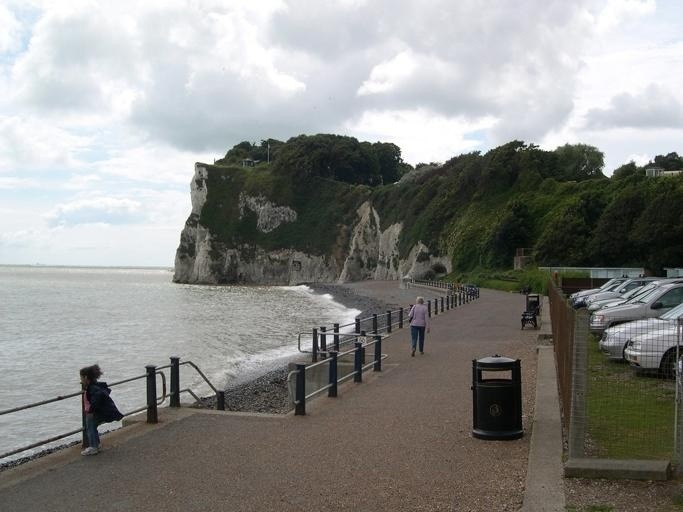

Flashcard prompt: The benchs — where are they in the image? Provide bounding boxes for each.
[521,304,543,329]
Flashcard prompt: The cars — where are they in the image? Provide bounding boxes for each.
[466,285,476,294]
[570,274,683,379]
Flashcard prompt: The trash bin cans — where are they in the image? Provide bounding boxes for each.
[526,294,540,316]
[471,355,525,441]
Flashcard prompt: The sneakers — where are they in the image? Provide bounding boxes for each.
[81,444,102,455]
[412,350,415,356]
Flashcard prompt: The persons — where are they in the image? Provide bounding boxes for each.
[407,296,430,358]
[77,363,124,458]
[445,280,465,300]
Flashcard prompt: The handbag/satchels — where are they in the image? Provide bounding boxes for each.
[409,316,413,323]
